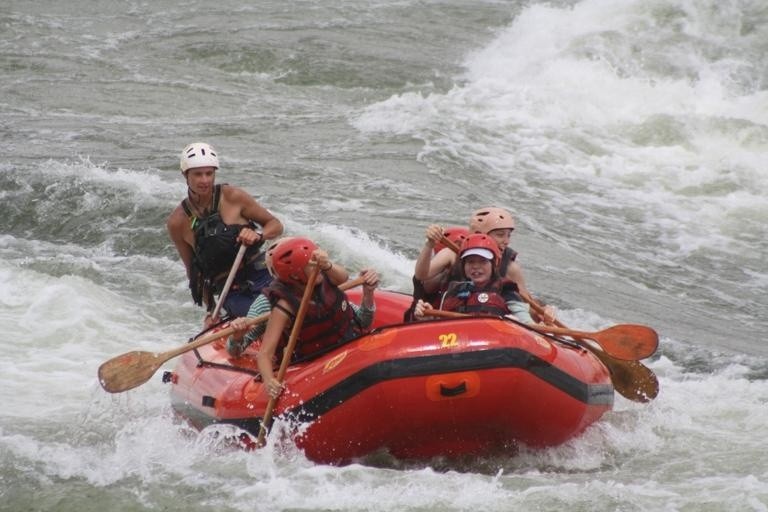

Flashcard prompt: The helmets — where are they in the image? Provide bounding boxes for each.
[265,237,318,286]
[434,208,514,264]
[180,143,218,172]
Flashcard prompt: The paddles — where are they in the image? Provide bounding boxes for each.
[434,233,658,403]
[415,307,660,361]
[97,277,364,393]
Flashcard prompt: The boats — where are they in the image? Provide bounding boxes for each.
[168,288,614,473]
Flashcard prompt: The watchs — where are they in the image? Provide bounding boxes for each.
[255,227,264,240]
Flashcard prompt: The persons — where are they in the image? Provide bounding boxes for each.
[415,208,556,326]
[256,237,369,399]
[226,237,380,363]
[403,228,470,324]
[410,233,536,328]
[168,142,283,329]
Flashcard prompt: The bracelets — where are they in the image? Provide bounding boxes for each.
[322,263,332,271]
